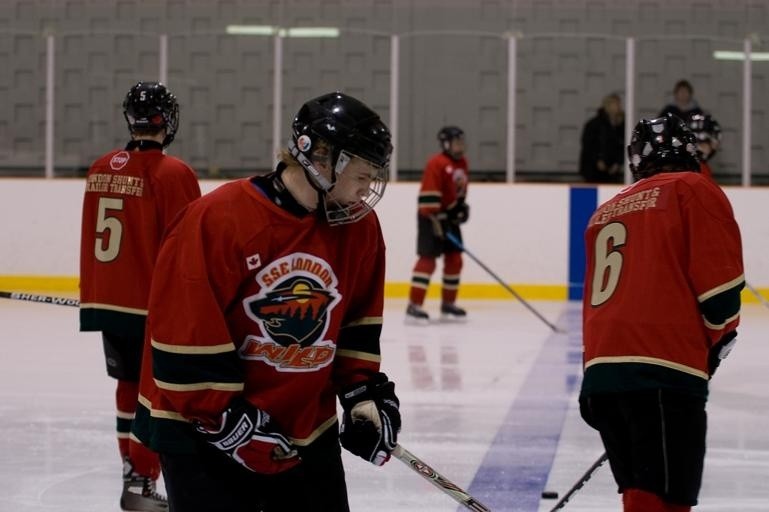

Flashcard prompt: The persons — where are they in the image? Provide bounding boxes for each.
[578,111,747,511]
[76,80,207,512]
[576,92,628,186]
[656,81,724,167]
[121,95,400,511]
[404,124,473,324]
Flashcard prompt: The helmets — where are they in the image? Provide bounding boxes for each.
[436,124,466,142]
[683,111,725,164]
[119,78,180,137]
[626,111,705,174]
[286,88,396,170]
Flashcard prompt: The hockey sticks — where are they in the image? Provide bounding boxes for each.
[444,227,573,334]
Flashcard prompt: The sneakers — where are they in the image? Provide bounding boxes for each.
[406,302,430,319]
[120,455,170,511]
[440,302,468,317]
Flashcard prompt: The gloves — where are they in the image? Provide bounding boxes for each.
[336,372,406,469]
[193,396,307,477]
[450,201,470,224]
[431,212,453,241]
